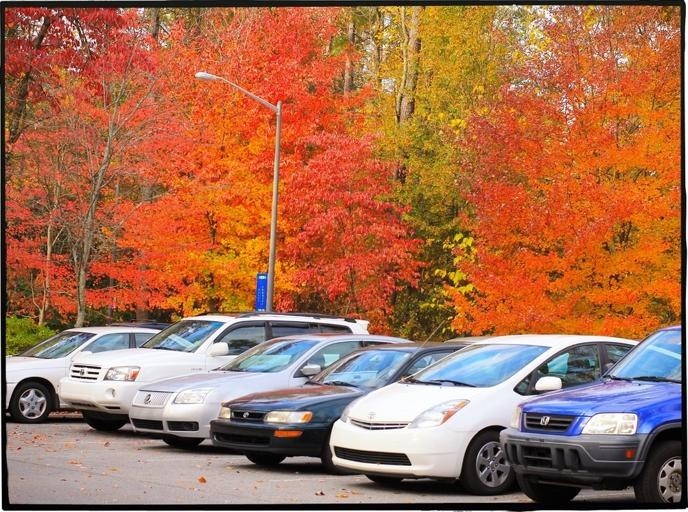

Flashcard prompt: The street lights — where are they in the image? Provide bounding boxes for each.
[195,72,282,313]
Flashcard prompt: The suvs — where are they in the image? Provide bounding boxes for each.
[498,323,682,503]
[59,316,369,431]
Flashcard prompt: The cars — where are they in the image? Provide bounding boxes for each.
[128,333,413,448]
[209,341,467,474]
[329,335,639,494]
[5,325,162,424]
